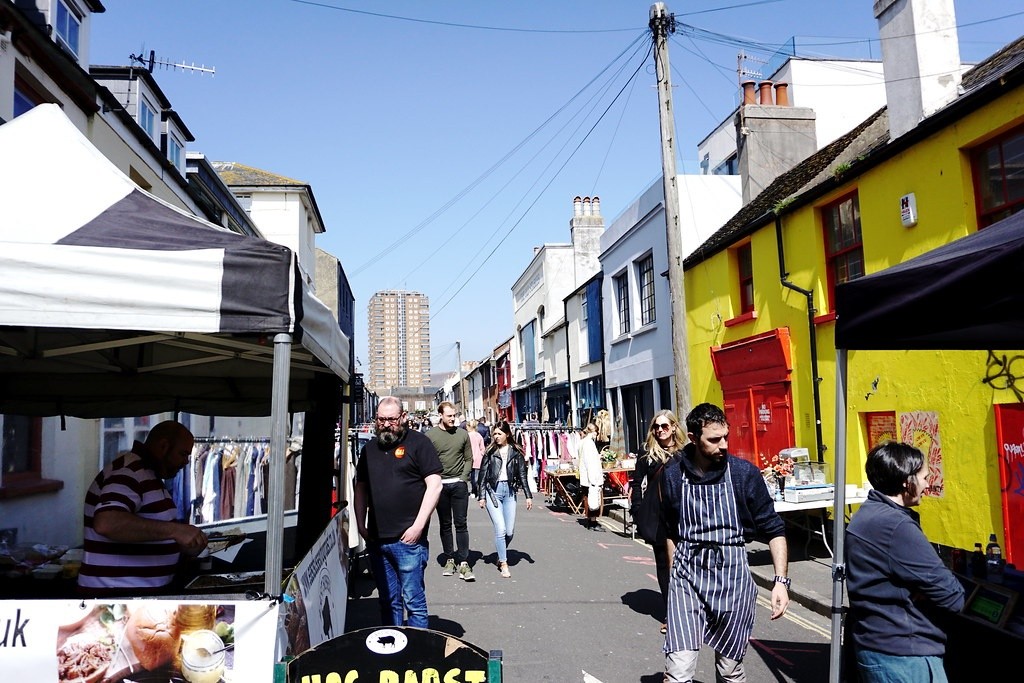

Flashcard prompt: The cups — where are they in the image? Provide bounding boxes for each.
[180,629,226,683]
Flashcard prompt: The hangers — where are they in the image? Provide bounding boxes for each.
[211,436,268,469]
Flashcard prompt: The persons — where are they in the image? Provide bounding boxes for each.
[352,397,444,628]
[843,440,966,683]
[632,409,693,632]
[579,425,607,532]
[477,420,533,577]
[77,420,209,598]
[406,413,434,434]
[423,402,475,580]
[658,403,789,683]
[457,414,491,500]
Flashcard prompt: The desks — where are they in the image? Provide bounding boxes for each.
[774,498,867,558]
[613,499,637,541]
[544,469,635,518]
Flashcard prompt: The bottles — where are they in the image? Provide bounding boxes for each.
[971,541,987,582]
[170,604,216,670]
[953,547,967,577]
[986,533,1002,583]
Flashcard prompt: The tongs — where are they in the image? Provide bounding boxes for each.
[207,532,242,541]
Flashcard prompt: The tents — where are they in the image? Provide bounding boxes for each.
[0,104,352,602]
[829,207,1023,683]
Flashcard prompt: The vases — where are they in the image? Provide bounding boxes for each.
[776,472,785,500]
[602,462,615,469]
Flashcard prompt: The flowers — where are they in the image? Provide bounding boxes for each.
[760,453,794,479]
[600,449,618,463]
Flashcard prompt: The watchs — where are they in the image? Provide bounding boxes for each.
[774,576,791,590]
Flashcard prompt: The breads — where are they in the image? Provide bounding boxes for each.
[126,609,179,671]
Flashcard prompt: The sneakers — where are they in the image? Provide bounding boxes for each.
[442,559,454,575]
[589,524,606,532]
[459,562,475,580]
[501,566,511,578]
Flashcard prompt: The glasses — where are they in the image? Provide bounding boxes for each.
[653,423,669,429]
[377,414,402,424]
